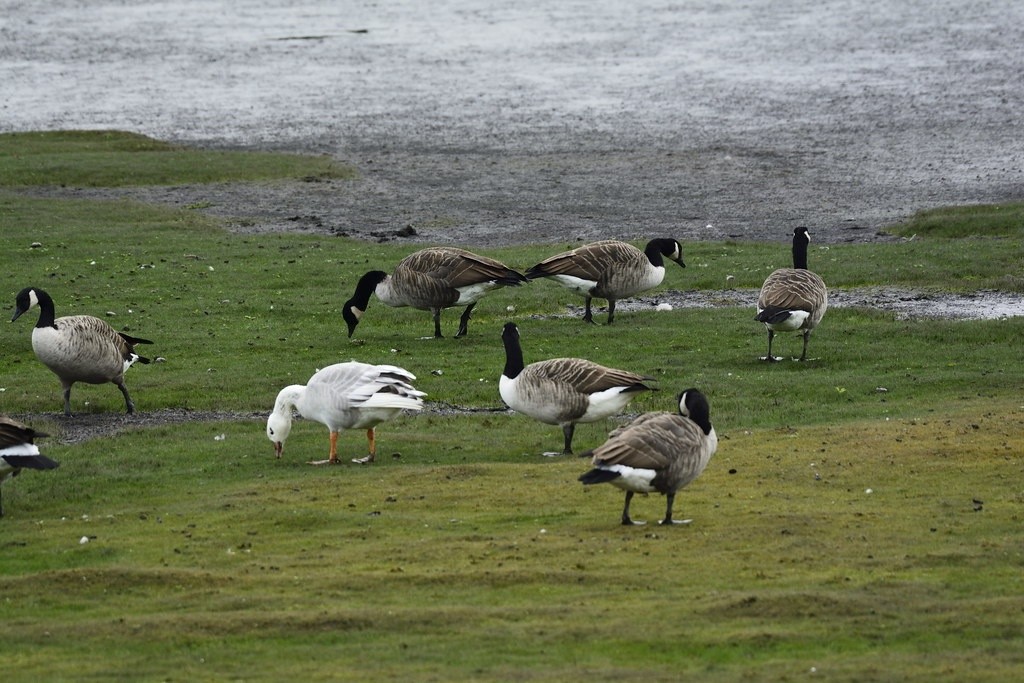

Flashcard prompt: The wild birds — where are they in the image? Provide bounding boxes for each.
[523,235,686,325]
[265,361,428,466]
[755,225,829,366]
[11,286,155,418]
[575,387,719,527]
[497,322,661,460]
[342,247,533,340]
[0,417,59,518]
